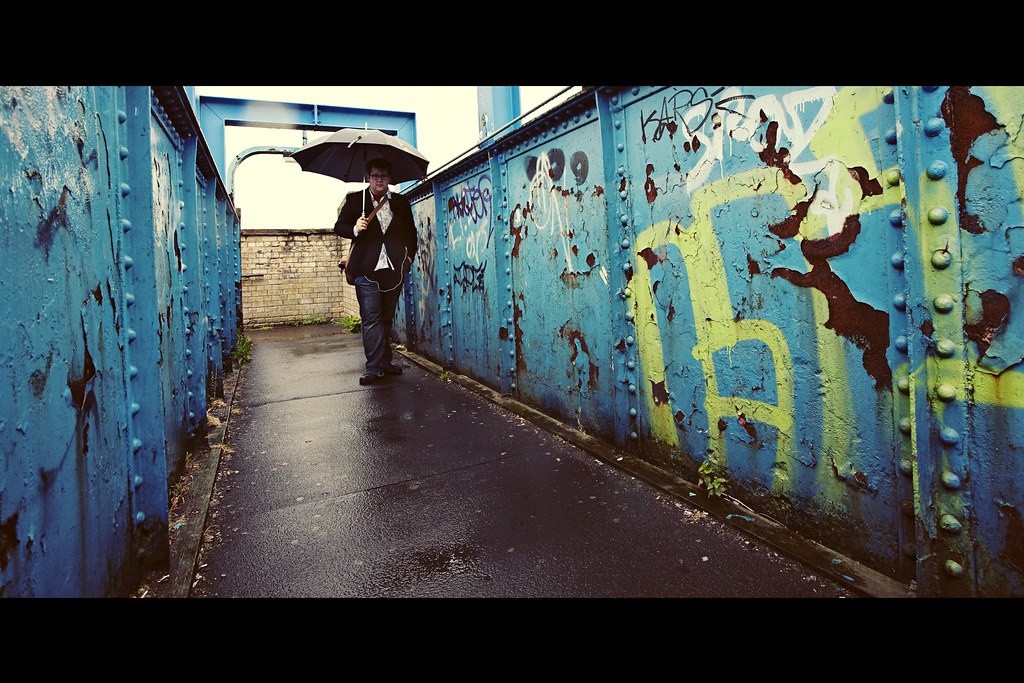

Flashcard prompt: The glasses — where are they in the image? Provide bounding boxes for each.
[369,174,391,180]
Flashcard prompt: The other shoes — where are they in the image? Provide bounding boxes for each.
[380,360,403,374]
[360,373,377,384]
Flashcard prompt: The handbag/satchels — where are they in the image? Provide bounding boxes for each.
[338,254,355,285]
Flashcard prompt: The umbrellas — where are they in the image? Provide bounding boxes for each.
[289,122,430,225]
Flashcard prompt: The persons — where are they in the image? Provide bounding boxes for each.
[332,157,419,386]
